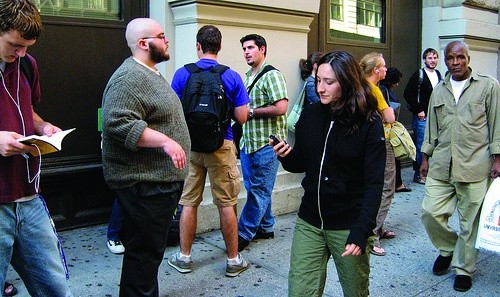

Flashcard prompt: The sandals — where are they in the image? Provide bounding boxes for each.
[380,230,395,239]
[4,281,18,296]
[369,244,386,256]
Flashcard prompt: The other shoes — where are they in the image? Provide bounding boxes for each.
[453,275,472,291]
[413,175,425,184]
[433,254,451,275]
[238,235,250,252]
[253,230,274,239]
[396,187,411,192]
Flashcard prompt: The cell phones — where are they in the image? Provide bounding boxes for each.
[269,134,288,152]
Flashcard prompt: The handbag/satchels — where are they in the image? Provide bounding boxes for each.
[475,176,500,254]
[390,122,416,161]
[288,82,308,133]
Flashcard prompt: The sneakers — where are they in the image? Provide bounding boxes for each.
[168,251,193,273]
[225,252,250,277]
[106,240,125,253]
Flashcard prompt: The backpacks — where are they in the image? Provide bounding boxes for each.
[181,62,229,154]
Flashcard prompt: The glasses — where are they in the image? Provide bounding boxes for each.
[142,34,165,39]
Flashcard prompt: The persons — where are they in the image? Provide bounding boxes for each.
[269,50,387,297]
[107,196,125,254]
[420,41,500,291]
[2,281,17,297]
[0,0,71,297]
[103,19,191,297]
[358,52,396,257]
[378,67,412,193]
[403,48,442,185]
[299,52,326,105]
[231,34,289,252]
[171,24,251,277]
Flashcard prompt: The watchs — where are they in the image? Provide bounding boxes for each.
[249,108,253,120]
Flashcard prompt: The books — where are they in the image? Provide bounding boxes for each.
[15,128,76,157]
